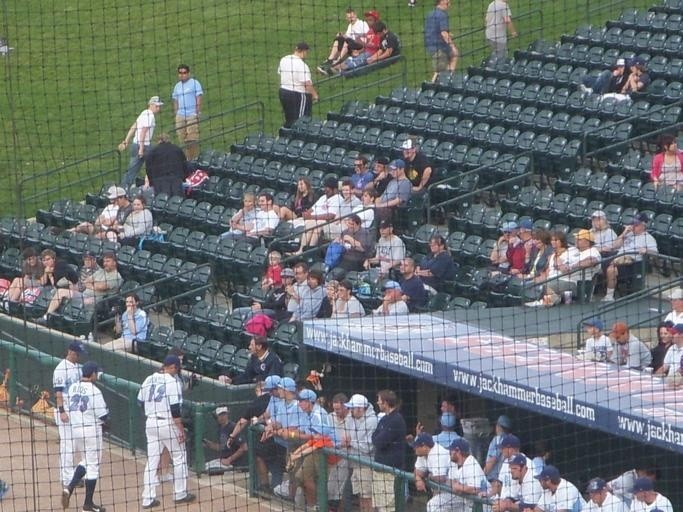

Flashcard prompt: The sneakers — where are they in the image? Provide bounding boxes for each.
[62,488,70,507]
[143,499,163,509]
[318,67,332,76]
[175,493,194,505]
[65,479,84,487]
[83,504,106,512]
[600,296,615,302]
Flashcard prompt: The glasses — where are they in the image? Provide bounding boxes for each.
[503,231,515,234]
[178,71,187,74]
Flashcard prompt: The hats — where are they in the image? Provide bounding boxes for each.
[591,210,606,218]
[516,221,534,230]
[365,10,379,19]
[496,435,520,448]
[277,378,296,391]
[533,466,560,481]
[69,340,86,353]
[440,413,455,427]
[399,139,414,149]
[609,322,628,338]
[215,407,228,416]
[297,390,317,402]
[81,361,102,376]
[583,320,603,329]
[669,323,683,335]
[629,57,645,65]
[669,288,683,300]
[163,354,181,364]
[344,394,368,408]
[408,433,434,447]
[149,96,164,106]
[586,477,606,492]
[500,223,518,232]
[108,188,126,200]
[280,268,295,278]
[380,281,400,292]
[615,58,628,66]
[388,160,405,169]
[261,375,281,390]
[503,455,525,464]
[496,415,512,427]
[532,458,544,474]
[378,220,392,229]
[627,212,648,222]
[575,229,594,241]
[626,479,654,493]
[445,439,468,453]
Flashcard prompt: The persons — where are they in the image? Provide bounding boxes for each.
[360,157,392,199]
[93,187,133,239]
[117,95,163,194]
[101,293,148,352]
[507,216,533,275]
[285,188,375,255]
[373,158,411,217]
[664,289,683,324]
[412,433,451,492]
[342,394,378,512]
[371,280,408,315]
[250,375,280,425]
[532,230,567,282]
[0,247,44,313]
[144,133,189,200]
[256,378,298,442]
[582,478,625,512]
[488,221,519,277]
[521,466,585,512]
[414,235,453,289]
[484,0,517,68]
[586,210,618,246]
[598,56,652,104]
[39,249,78,290]
[230,268,295,319]
[65,185,120,235]
[545,229,601,295]
[67,251,101,292]
[651,320,675,377]
[224,381,268,448]
[278,270,327,326]
[482,416,515,480]
[172,63,203,165]
[651,133,683,193]
[319,8,369,66]
[591,58,625,94]
[497,436,533,497]
[414,411,464,446]
[653,323,683,378]
[268,263,308,320]
[330,281,365,320]
[287,422,347,512]
[316,9,381,74]
[218,336,284,384]
[276,389,328,439]
[399,138,432,191]
[330,20,399,79]
[285,177,343,243]
[427,438,490,512]
[105,195,153,242]
[260,250,281,300]
[598,210,658,304]
[581,318,611,360]
[52,341,87,488]
[371,389,406,512]
[216,191,257,244]
[608,323,653,370]
[137,353,196,509]
[326,391,350,449]
[516,226,556,280]
[350,156,374,190]
[312,280,339,321]
[491,455,543,511]
[337,180,361,216]
[273,177,314,221]
[275,41,319,141]
[397,257,425,310]
[435,395,465,435]
[31,252,123,327]
[627,476,650,512]
[325,214,369,280]
[238,192,278,249]
[362,219,406,277]
[607,460,650,497]
[422,0,458,84]
[61,363,109,512]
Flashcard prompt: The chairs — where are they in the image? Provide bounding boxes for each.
[0,3,682,379]
